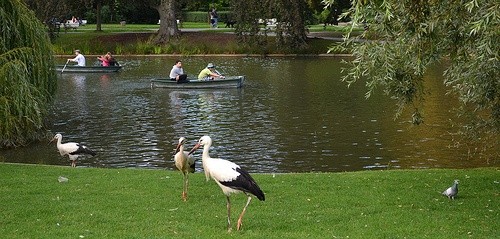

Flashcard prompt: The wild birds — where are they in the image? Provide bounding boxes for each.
[187,134,267,236]
[173,137,197,202]
[47,134,100,168]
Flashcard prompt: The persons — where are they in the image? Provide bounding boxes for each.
[169,61,188,81]
[97,52,120,67]
[71,16,80,30]
[210,7,219,28]
[67,50,86,67]
[198,63,225,80]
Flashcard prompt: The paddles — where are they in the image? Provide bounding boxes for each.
[214,69,225,80]
[60,59,70,73]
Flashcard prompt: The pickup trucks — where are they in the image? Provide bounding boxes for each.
[55,20,79,30]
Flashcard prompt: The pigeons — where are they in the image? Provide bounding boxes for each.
[441,179,459,201]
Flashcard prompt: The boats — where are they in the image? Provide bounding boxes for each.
[56,64,125,72]
[150,74,246,90]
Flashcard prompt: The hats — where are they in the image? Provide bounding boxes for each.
[207,63,214,68]
